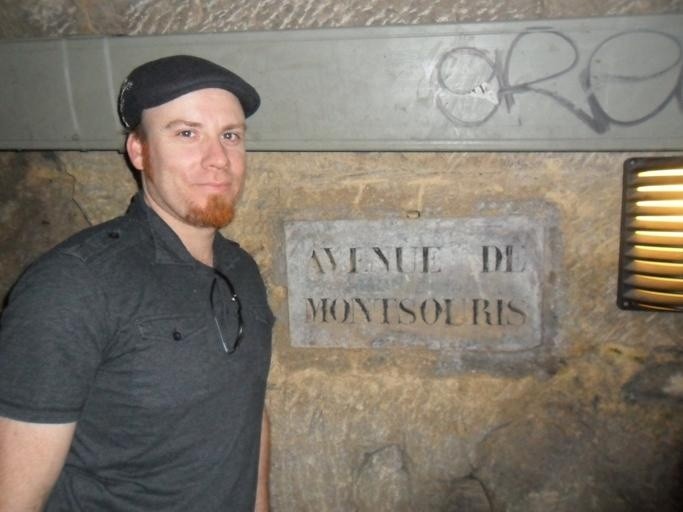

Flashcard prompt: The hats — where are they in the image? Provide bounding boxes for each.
[118,55,260,130]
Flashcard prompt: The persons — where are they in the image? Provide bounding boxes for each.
[0,54,276,512]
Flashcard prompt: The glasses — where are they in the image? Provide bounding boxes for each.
[210,269,245,355]
